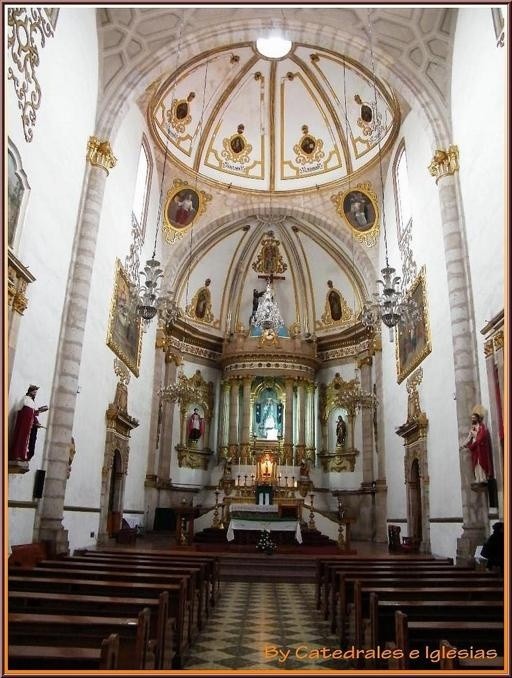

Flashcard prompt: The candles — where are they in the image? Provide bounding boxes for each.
[237,456,295,478]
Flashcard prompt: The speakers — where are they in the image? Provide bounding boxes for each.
[33,469,46,499]
[487,478,498,508]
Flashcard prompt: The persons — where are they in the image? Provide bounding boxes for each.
[322,280,351,324]
[186,279,214,323]
[460,414,494,483]
[249,288,266,325]
[187,408,205,439]
[174,193,196,224]
[336,416,346,444]
[480,522,503,571]
[10,386,41,461]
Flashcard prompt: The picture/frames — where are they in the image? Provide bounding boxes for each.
[392,263,433,385]
[104,256,143,378]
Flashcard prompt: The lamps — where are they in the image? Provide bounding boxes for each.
[251,276,284,338]
[327,53,377,424]
[156,41,211,413]
[360,21,419,344]
[120,16,184,330]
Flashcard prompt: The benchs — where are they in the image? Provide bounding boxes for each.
[309,553,504,669]
[6,541,220,669]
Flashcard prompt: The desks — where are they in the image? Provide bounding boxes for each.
[224,517,304,545]
